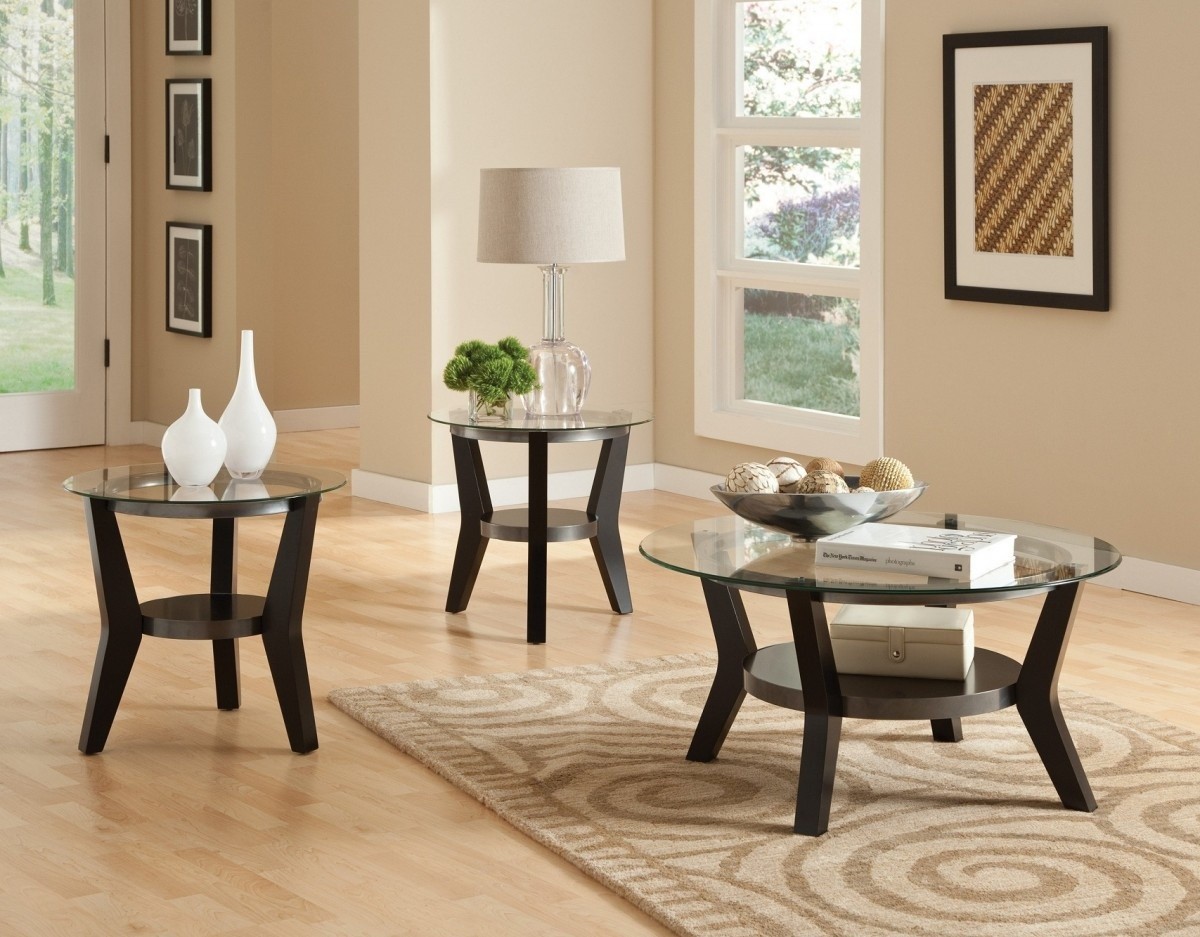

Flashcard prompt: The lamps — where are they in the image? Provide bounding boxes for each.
[476,167,625,416]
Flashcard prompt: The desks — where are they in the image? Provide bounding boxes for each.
[641,511,1122,837]
[63,463,347,754]
[427,407,653,643]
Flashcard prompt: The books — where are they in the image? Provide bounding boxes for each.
[815,522,1017,580]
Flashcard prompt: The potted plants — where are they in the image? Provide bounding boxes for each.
[444,337,542,422]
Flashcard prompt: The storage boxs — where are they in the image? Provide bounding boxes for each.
[829,605,975,680]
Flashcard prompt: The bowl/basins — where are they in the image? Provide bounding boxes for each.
[711,477,930,543]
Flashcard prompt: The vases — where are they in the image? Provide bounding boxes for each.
[217,329,276,480]
[162,388,228,487]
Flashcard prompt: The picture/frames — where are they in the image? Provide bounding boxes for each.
[165,0,212,56]
[166,221,212,338]
[165,78,212,192]
[943,26,1108,311]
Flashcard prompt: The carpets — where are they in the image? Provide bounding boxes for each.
[328,650,1200,937]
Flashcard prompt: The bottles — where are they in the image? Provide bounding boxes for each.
[168,487,219,502]
[161,388,226,487]
[217,330,277,480]
[220,481,270,501]
